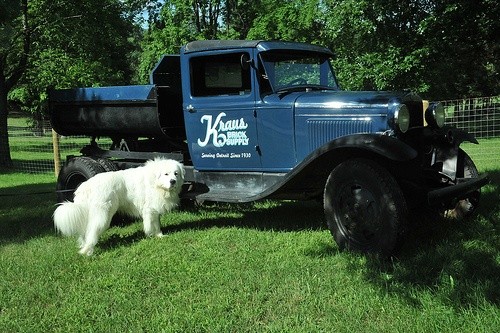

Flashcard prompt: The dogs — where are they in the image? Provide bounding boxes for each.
[49,155,186,258]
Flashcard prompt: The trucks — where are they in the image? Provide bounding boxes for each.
[48,40,491,264]
